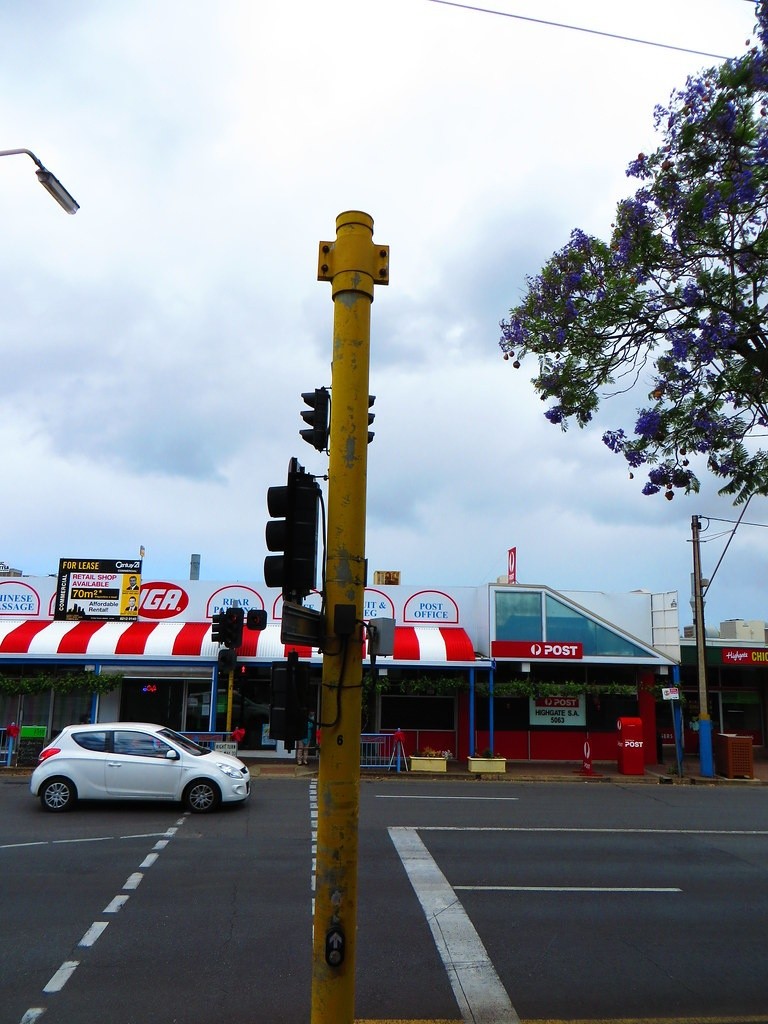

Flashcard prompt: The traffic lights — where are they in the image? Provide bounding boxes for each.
[247,610,266,630]
[237,664,247,675]
[368,394,375,444]
[298,388,329,452]
[225,608,244,648]
[212,613,226,644]
[265,457,321,603]
[218,649,237,671]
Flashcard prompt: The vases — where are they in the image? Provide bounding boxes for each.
[468,756,506,773]
[409,755,446,772]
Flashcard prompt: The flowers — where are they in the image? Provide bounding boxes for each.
[472,748,504,759]
[413,745,453,760]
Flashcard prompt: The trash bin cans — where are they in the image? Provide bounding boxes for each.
[616,717,644,775]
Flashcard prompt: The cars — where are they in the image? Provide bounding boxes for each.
[30,722,251,814]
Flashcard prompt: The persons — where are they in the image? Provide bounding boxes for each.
[126,576,139,590]
[125,597,137,611]
